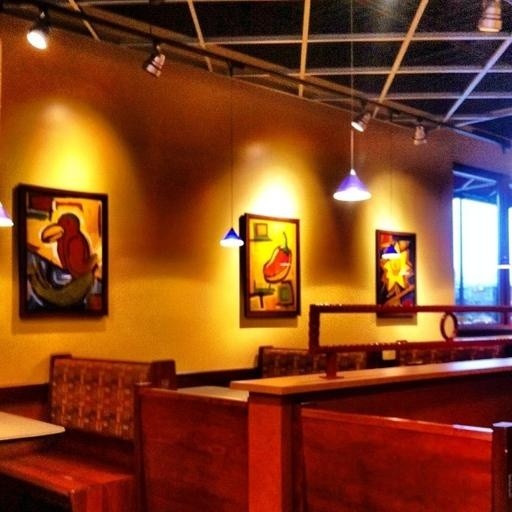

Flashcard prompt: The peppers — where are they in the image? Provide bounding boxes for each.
[263,232,292,284]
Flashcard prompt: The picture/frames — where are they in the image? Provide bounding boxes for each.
[240,212,301,319]
[374,228,418,318]
[13,182,108,317]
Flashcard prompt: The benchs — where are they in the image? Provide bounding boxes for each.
[0,343,512,512]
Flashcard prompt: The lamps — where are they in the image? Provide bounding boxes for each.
[475,0,503,33]
[0,200,15,227]
[219,76,245,247]
[27,8,50,50]
[333,0,372,202]
[412,123,427,145]
[351,99,372,132]
[142,42,166,76]
